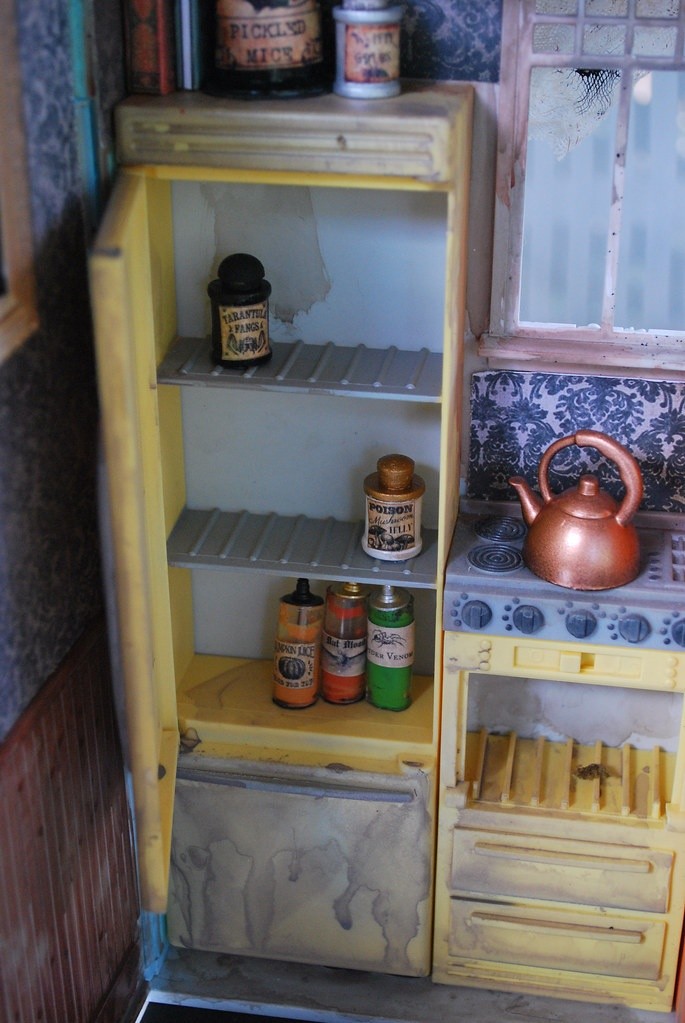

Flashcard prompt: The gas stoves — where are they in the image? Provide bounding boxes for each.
[443,514,685,653]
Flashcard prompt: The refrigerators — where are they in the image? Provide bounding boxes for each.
[85,81,478,979]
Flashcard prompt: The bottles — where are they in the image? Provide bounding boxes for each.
[272,579,416,710]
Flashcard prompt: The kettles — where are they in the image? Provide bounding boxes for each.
[507,431,642,590]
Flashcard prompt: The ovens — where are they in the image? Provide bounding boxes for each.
[436,631,685,1013]
